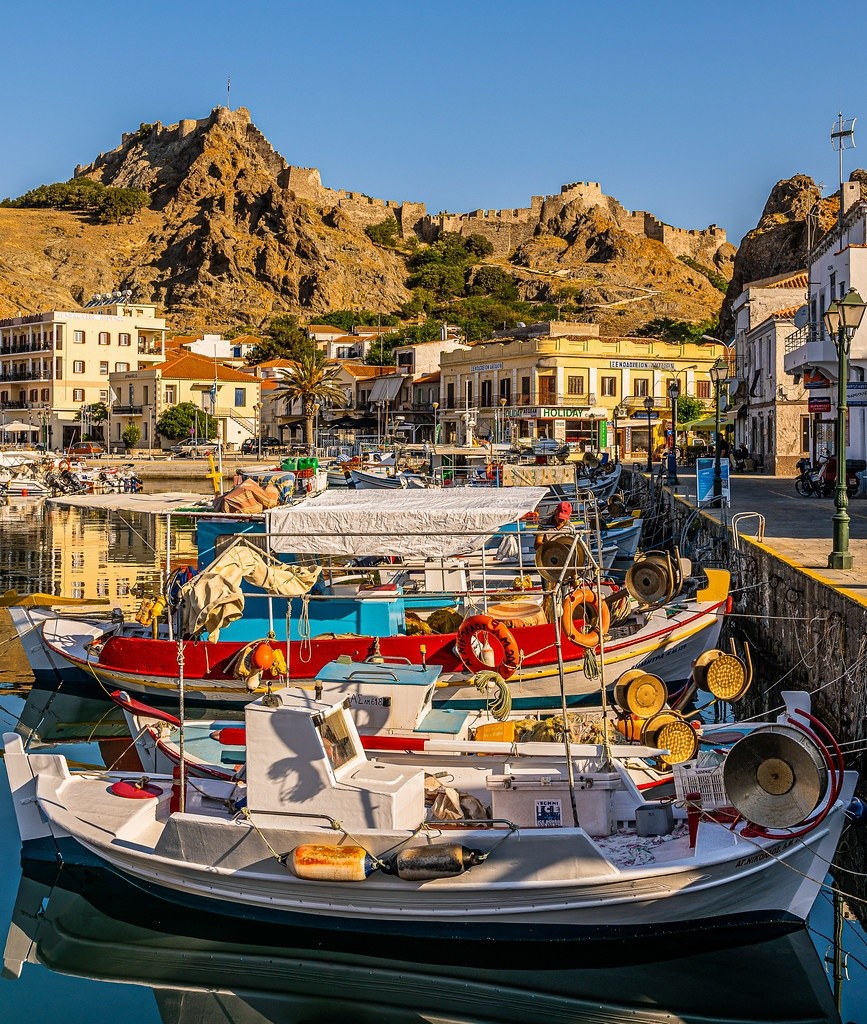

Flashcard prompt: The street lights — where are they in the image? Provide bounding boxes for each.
[710,359,729,508]
[823,287,866,570]
[644,397,654,472]
[667,382,681,456]
[613,406,621,465]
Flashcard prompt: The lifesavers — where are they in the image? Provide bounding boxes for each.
[457,615,519,680]
[561,590,610,648]
[486,464,502,480]
[59,460,70,474]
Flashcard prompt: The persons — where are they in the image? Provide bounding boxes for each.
[720,433,729,458]
[739,443,748,459]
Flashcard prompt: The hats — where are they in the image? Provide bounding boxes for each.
[556,501,572,520]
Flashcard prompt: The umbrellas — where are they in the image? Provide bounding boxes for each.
[675,417,734,453]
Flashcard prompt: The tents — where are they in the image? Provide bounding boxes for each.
[320,416,361,447]
[356,418,385,443]
[278,416,332,448]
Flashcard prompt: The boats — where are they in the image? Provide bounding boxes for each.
[2,417,865,1017]
